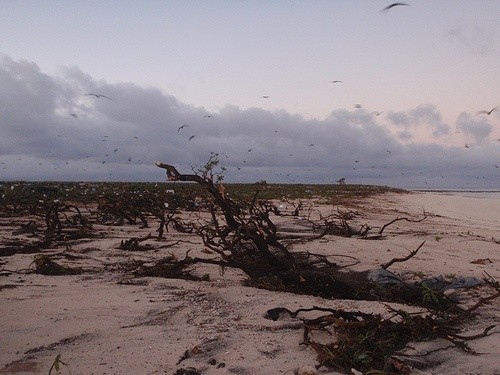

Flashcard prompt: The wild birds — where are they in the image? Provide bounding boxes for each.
[380,3,410,12]
[2,80,500,191]
